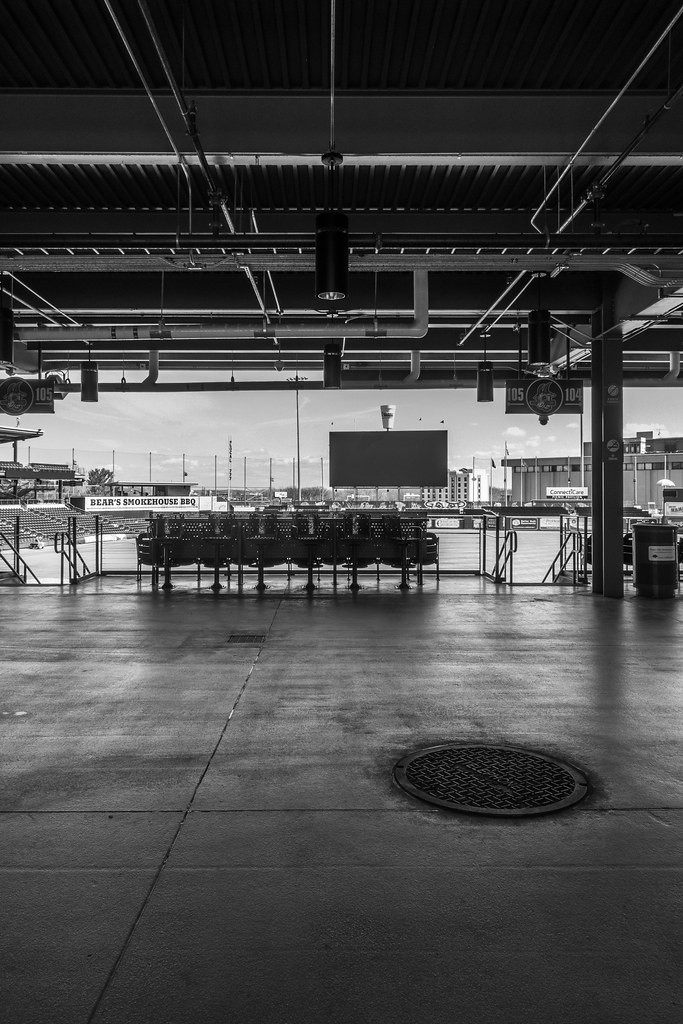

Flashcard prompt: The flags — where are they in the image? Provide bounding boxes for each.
[492,459,496,468]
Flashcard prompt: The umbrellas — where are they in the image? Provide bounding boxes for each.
[657,479,675,487]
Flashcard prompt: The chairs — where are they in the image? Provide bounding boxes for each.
[135,516,439,591]
[585,533,683,583]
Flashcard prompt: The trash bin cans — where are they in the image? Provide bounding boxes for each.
[630,522,679,598]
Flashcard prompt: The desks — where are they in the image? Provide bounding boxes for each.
[145,518,429,589]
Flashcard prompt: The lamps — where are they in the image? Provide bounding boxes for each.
[527,272,551,365]
[45,370,70,400]
[0,270,14,364]
[323,312,341,388]
[81,341,98,402]
[477,333,493,403]
[315,150,350,300]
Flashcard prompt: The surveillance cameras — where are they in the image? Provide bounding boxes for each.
[274,361,286,371]
